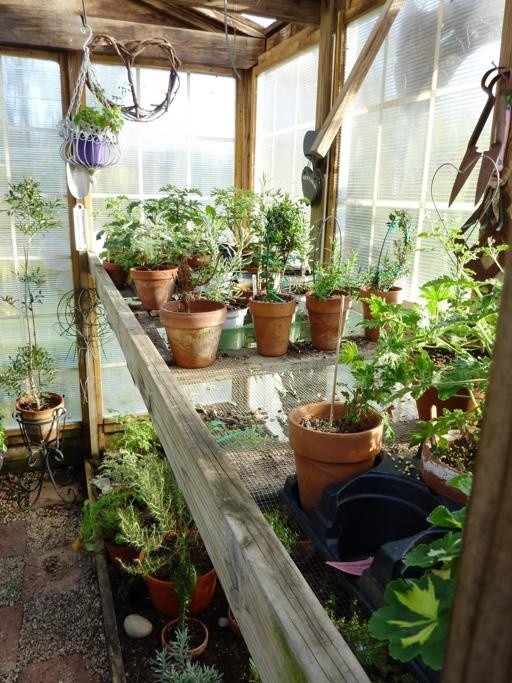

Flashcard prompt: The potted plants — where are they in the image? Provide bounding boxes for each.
[96,186,436,363]
[2,183,62,454]
[64,99,124,167]
[286,240,508,518]
[78,413,216,655]
